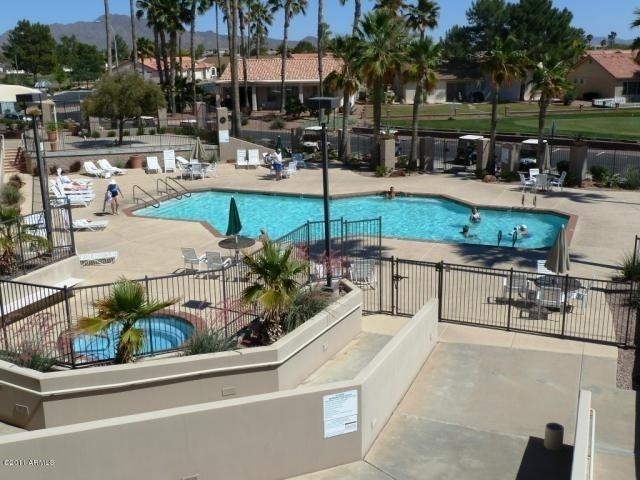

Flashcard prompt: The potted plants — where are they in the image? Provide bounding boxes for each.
[45,120,57,140]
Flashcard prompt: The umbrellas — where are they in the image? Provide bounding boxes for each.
[539,141,551,174]
[190,137,207,160]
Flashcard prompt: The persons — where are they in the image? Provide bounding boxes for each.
[104,179,126,218]
[468,205,483,224]
[460,223,472,238]
[385,185,396,200]
[271,148,286,180]
[512,224,532,239]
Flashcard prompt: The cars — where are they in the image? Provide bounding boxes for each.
[3,112,22,120]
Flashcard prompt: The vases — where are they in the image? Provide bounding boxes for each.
[130,155,141,168]
[72,125,77,136]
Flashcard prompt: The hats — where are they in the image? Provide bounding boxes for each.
[110,180,117,184]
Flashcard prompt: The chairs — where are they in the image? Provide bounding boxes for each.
[536,286,562,310]
[11,158,125,263]
[503,272,527,299]
[518,168,567,192]
[575,277,595,314]
[536,259,557,284]
[232,149,306,169]
[180,247,206,279]
[147,149,205,181]
[205,252,232,279]
[345,260,375,290]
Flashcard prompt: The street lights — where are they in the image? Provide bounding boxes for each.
[96,20,118,69]
[11,31,25,70]
[20,113,54,257]
[307,97,340,287]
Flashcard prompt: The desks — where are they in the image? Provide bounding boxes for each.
[534,275,580,303]
[218,237,255,260]
